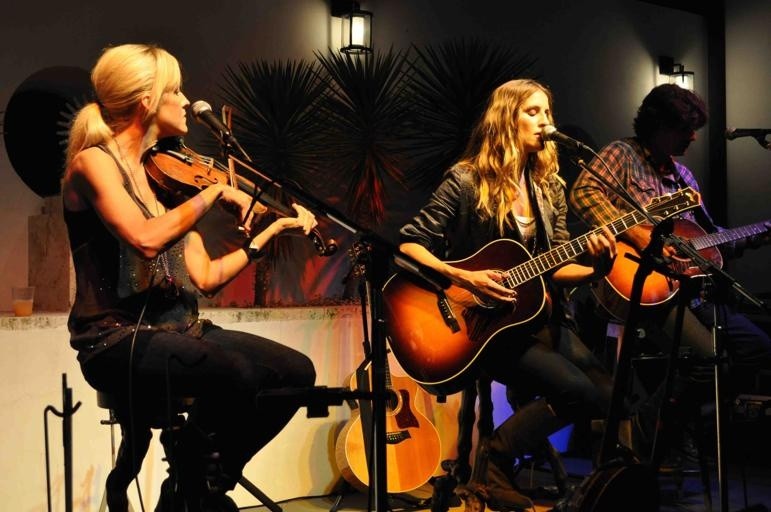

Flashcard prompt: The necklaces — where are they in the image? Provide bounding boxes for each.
[115,137,174,284]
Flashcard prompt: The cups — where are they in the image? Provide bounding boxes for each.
[11,287,35,316]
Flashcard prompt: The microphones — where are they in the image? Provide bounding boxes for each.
[190,99,234,144]
[724,127,771,140]
[542,125,584,149]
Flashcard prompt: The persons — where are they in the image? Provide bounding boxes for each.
[570,81,771,476]
[394,75,619,512]
[57,41,321,510]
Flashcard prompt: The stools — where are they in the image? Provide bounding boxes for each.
[451,336,712,512]
[95,388,197,512]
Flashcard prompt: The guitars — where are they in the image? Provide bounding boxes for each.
[335,240,441,495]
[588,218,770,325]
[382,185,701,395]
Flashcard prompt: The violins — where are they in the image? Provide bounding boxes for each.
[144,141,338,258]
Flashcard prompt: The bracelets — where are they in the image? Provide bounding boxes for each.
[241,239,264,264]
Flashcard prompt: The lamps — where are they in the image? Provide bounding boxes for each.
[654,54,695,92]
[328,0,376,56]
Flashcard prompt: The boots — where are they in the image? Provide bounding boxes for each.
[479,396,561,510]
[677,347,713,464]
[636,366,681,447]
